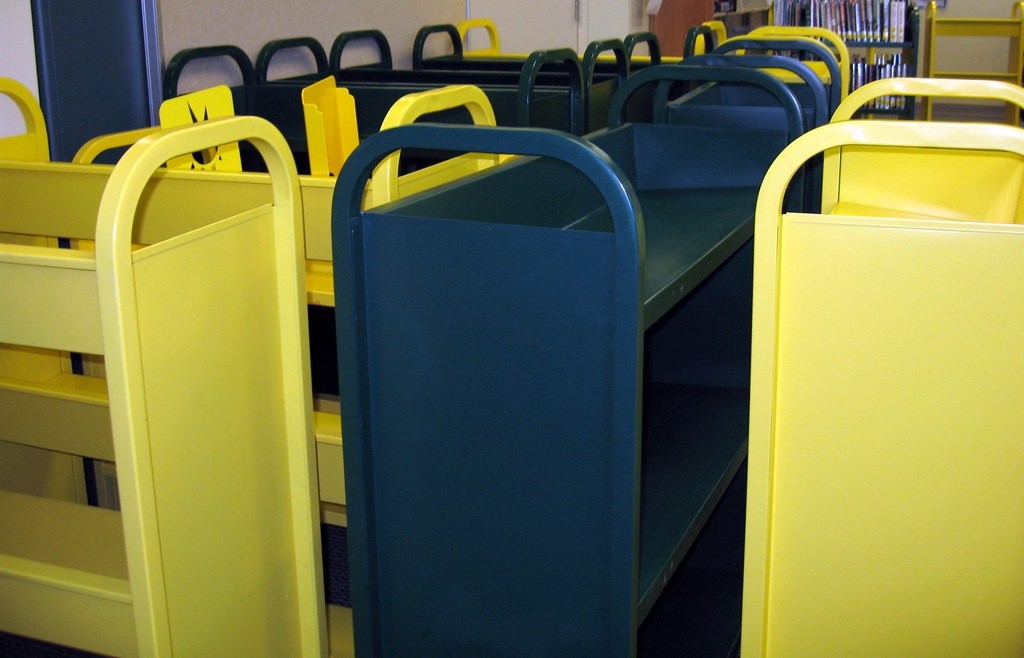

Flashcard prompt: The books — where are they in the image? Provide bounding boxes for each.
[770,49,907,110]
[770,0,906,44]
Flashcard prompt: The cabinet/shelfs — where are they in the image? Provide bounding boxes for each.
[0,0,1024,658]
